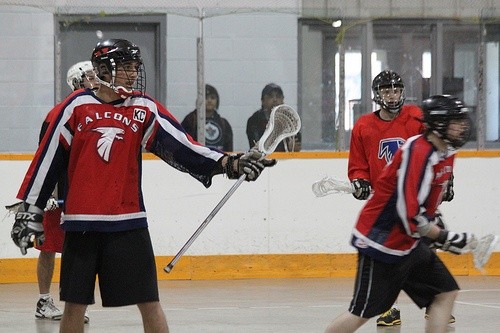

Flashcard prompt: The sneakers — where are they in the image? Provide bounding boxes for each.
[34,297,63,319]
[82,315,88,322]
[423,308,454,323]
[376,308,401,326]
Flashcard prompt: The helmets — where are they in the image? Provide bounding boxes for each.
[65,61,96,92]
[89,39,146,101]
[372,71,403,113]
[423,96,468,148]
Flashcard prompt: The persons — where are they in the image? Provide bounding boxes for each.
[348,70,456,326]
[246,83,302,153]
[324,94,497,333]
[181,84,233,152]
[11,38,276,333]
[35,60,103,323]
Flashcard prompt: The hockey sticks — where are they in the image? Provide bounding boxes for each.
[5,199,64,211]
[164,104,302,273]
[313,177,374,198]
[472,233,499,267]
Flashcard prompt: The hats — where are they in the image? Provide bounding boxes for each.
[262,83,284,97]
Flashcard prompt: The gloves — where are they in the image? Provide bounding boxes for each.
[432,229,471,253]
[10,202,45,254]
[351,178,370,200]
[226,148,276,182]
